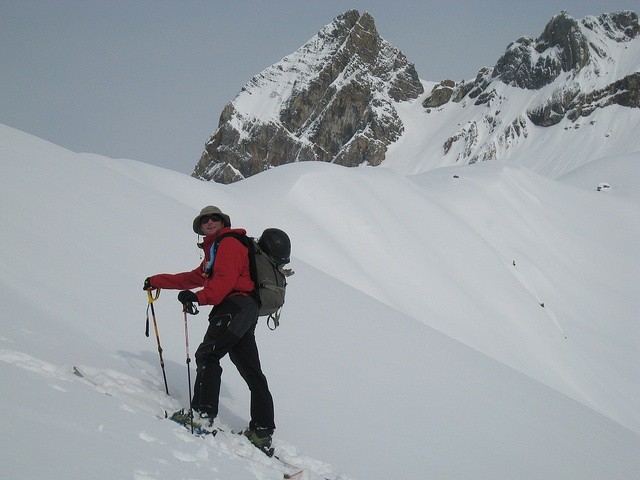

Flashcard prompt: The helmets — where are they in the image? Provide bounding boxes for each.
[258,227,291,264]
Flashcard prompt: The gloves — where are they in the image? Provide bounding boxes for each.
[142,276,156,291]
[277,261,294,278]
[178,289,199,306]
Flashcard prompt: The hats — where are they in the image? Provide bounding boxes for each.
[192,205,231,236]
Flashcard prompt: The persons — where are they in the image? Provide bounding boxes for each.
[142,204,277,451]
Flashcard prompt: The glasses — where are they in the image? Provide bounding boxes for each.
[200,215,222,224]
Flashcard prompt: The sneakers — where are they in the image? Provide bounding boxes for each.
[171,407,214,430]
[230,427,273,452]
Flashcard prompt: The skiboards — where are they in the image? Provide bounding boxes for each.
[74,366,330,480]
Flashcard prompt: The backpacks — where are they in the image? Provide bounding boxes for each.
[202,232,287,330]
